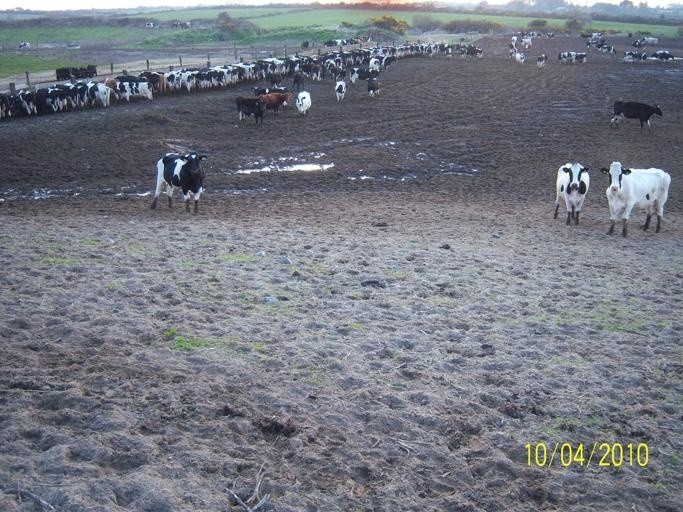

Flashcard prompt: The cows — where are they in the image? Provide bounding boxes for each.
[609,99,663,134]
[1,32,484,124]
[555,161,591,227]
[599,161,673,236]
[505,28,681,72]
[152,150,208,214]
